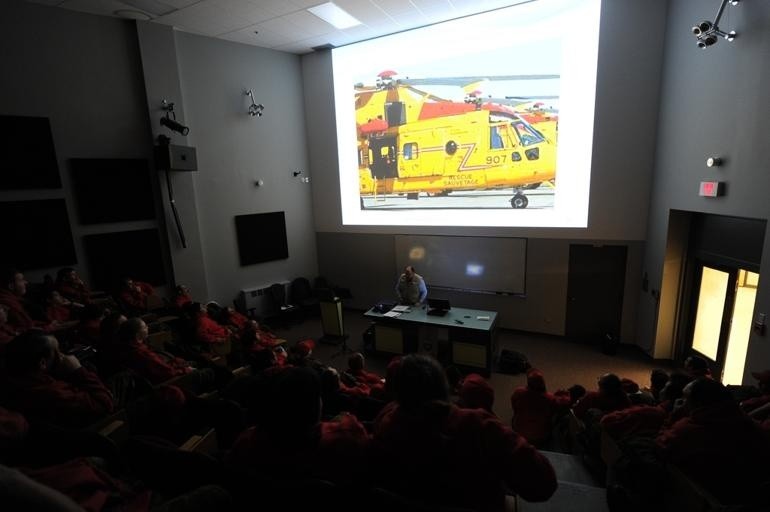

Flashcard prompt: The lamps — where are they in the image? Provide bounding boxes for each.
[158,102,191,137]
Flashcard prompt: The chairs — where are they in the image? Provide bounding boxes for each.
[233,276,344,330]
[1,284,769,512]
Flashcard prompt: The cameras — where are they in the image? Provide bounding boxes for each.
[68,345,97,367]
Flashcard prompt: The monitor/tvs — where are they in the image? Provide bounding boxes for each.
[427,298,451,317]
[235,211,289,267]
[0,115,62,188]
[80,227,176,290]
[71,157,155,225]
[0,198,79,271]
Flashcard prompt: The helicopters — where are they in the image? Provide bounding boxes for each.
[353,71,556,209]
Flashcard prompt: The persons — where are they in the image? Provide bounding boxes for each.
[364,354,569,511]
[367,77,555,113]
[575,355,770,512]
[1,265,388,511]
[395,266,427,307]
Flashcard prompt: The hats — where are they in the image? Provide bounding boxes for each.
[458,375,483,391]
[295,339,314,358]
[527,369,546,388]
[686,355,707,370]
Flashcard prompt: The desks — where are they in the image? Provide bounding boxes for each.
[364,300,500,380]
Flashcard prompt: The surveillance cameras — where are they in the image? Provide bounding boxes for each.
[692,21,719,49]
